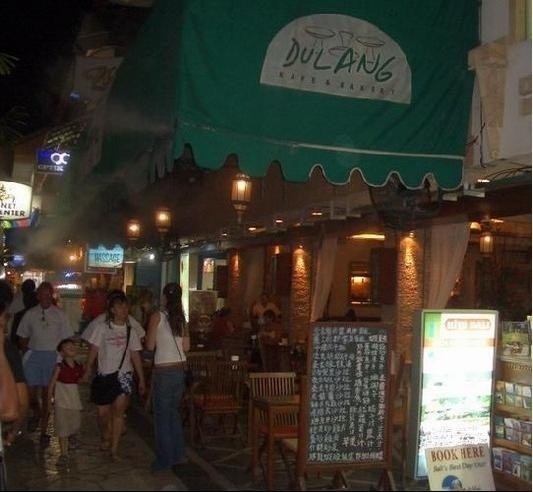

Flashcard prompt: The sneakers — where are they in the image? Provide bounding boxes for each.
[28,415,191,469]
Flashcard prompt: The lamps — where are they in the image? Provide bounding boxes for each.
[154,202,172,242]
[230,171,253,225]
[125,216,140,248]
[478,223,494,260]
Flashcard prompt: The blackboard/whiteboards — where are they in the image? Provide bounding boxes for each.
[298,322,391,472]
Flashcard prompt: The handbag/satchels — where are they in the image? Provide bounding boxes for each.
[184,366,194,388]
[88,371,122,407]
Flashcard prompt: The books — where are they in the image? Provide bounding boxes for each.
[491,379,531,483]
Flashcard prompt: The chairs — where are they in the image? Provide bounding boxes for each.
[181,325,414,491]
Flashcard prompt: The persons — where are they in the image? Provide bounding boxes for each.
[0,274,191,477]
[191,291,359,373]
